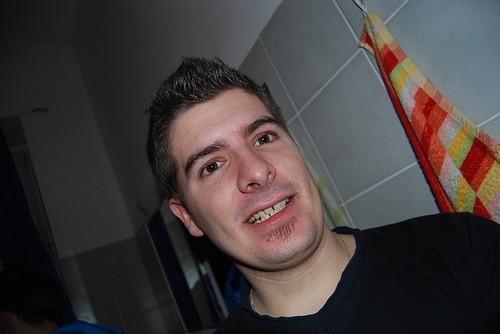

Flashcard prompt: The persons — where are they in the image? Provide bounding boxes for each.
[146,56,500,334]
[0,253,126,334]
